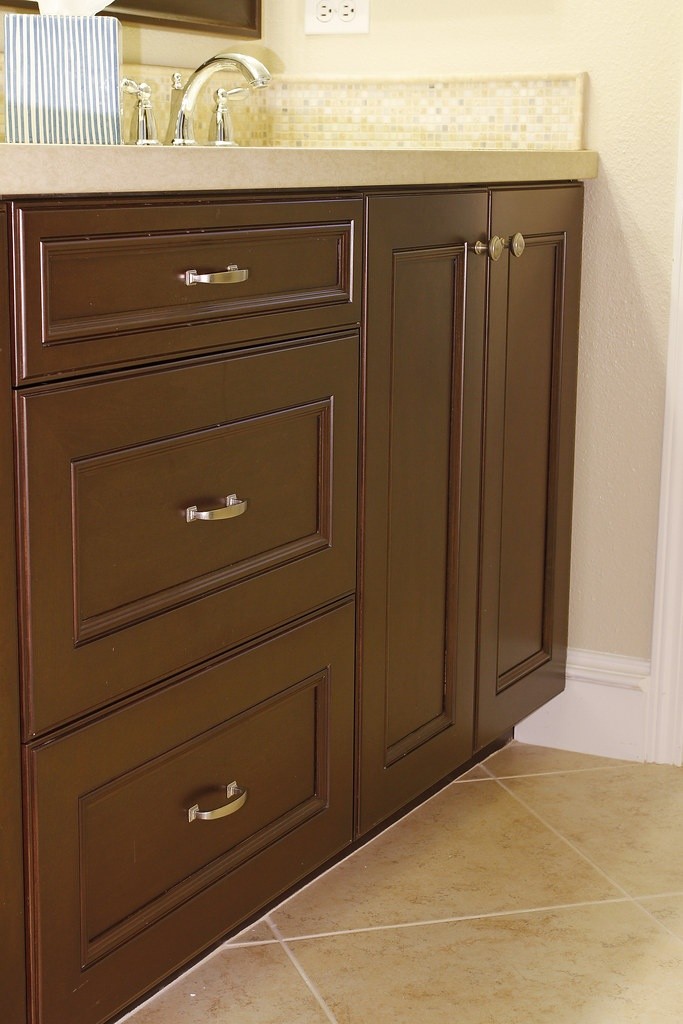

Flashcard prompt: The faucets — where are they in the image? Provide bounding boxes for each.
[171,50,274,146]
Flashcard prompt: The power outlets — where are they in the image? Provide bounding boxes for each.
[305,0,369,36]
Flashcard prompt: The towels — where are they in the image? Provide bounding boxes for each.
[3,11,124,146]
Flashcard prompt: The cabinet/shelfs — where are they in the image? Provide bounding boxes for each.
[0,178,586,1024]
